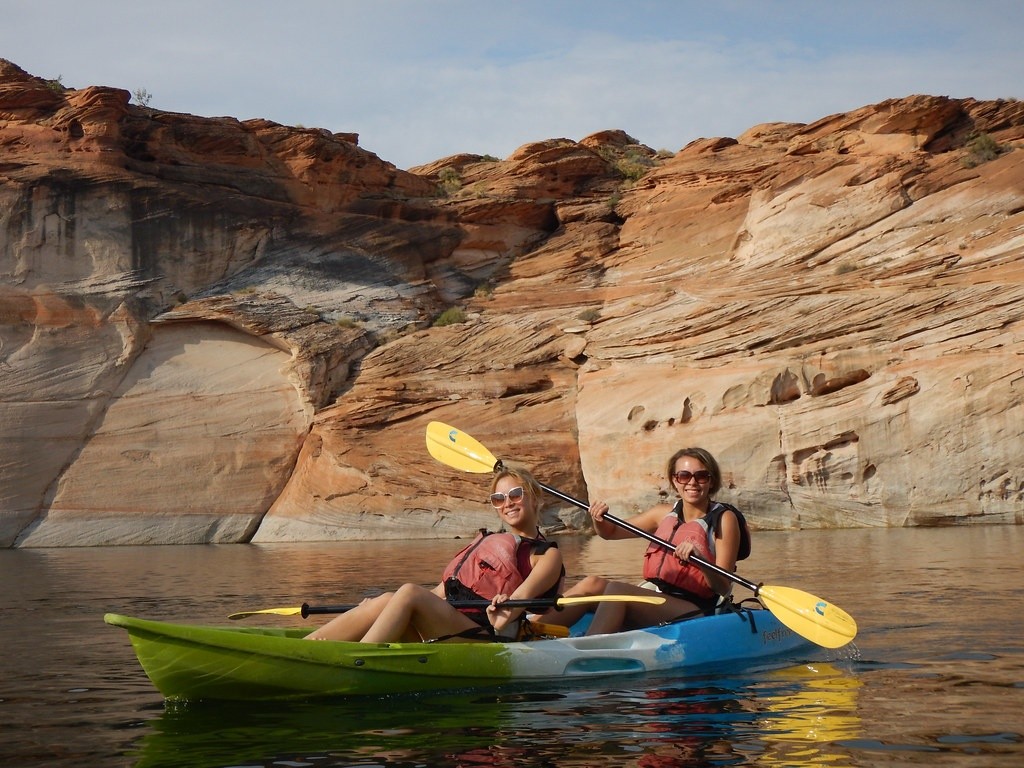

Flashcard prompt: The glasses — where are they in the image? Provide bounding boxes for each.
[489,486,526,508]
[672,470,711,484]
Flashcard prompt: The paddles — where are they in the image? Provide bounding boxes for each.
[426,419,857,649]
[226,594,666,620]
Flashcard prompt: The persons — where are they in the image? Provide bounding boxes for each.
[301,447,741,642]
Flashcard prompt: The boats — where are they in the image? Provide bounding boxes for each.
[102,597,821,703]
